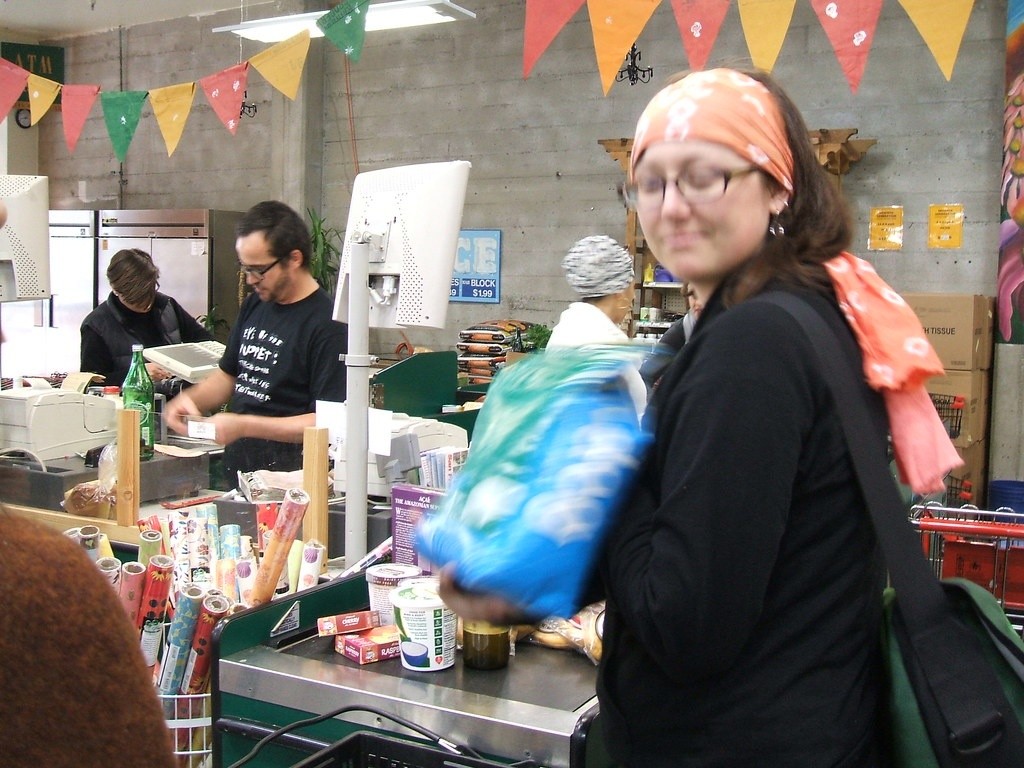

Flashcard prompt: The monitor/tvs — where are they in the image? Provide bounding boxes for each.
[333,160,470,328]
[142,342,224,384]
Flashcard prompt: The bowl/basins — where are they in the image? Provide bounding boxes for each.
[401,642,428,666]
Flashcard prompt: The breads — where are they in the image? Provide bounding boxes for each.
[456,601,608,661]
[62,480,117,522]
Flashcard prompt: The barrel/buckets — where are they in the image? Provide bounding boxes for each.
[989,480,1024,523]
[366,563,422,625]
[390,583,457,671]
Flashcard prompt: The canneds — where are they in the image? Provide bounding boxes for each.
[463,618,511,670]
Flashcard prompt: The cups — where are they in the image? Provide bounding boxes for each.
[640,307,648,321]
[463,620,510,669]
[649,308,657,321]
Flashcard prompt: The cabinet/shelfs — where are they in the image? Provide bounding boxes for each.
[635,282,684,328]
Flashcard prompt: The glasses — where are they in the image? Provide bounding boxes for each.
[153,280,160,295]
[622,164,758,212]
[239,252,291,280]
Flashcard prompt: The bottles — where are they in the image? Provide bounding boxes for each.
[511,325,523,353]
[121,344,155,460]
[103,386,120,399]
[633,333,664,344]
[644,263,654,283]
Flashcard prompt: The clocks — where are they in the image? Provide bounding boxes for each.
[14,108,32,129]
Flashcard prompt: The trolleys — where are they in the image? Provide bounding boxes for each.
[908,393,1024,634]
[226,705,536,768]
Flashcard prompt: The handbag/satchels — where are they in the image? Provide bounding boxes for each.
[883,574,1023,768]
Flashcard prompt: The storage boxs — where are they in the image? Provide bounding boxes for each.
[318,612,381,637]
[392,482,454,577]
[335,625,401,665]
[900,293,997,521]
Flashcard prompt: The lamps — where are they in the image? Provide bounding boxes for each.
[212,0,476,43]
[239,0,257,118]
[614,41,654,85]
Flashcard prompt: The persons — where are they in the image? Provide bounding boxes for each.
[438,67,911,768]
[80,249,219,449]
[162,200,349,500]
[545,234,668,349]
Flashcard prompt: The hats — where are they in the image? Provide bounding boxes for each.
[563,236,637,298]
